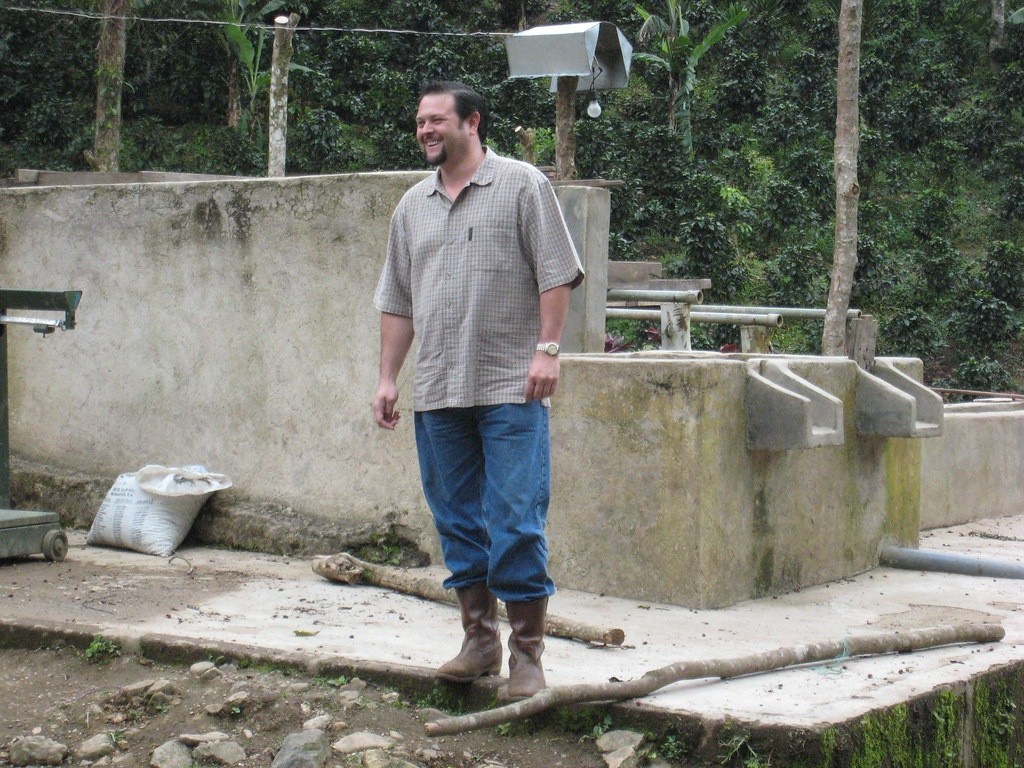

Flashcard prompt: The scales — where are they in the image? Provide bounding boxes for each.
[0,287,87,563]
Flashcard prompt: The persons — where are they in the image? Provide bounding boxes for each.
[372,81,586,700]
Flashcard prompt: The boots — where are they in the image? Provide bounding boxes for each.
[504,595,547,701]
[435,580,504,683]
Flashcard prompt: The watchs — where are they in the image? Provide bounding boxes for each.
[536,343,560,356]
